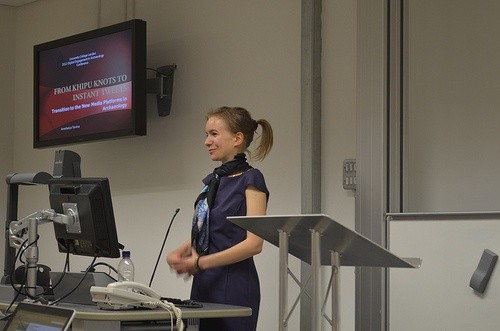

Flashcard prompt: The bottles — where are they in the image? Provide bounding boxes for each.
[118,251,134,291]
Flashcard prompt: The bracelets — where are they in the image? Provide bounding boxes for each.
[197,255,203,272]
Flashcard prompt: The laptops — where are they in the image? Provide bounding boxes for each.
[2,301,76,331]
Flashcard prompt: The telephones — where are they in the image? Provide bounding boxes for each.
[89,281,162,310]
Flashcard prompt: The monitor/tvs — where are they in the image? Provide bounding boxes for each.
[48,177,121,259]
[34,19,147,149]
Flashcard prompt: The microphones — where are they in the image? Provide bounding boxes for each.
[149,208,180,287]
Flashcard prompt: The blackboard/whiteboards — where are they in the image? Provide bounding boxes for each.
[384,211,500,331]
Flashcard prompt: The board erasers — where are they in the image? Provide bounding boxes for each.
[468,247,499,295]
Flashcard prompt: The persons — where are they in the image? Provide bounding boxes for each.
[167,106,274,331]
